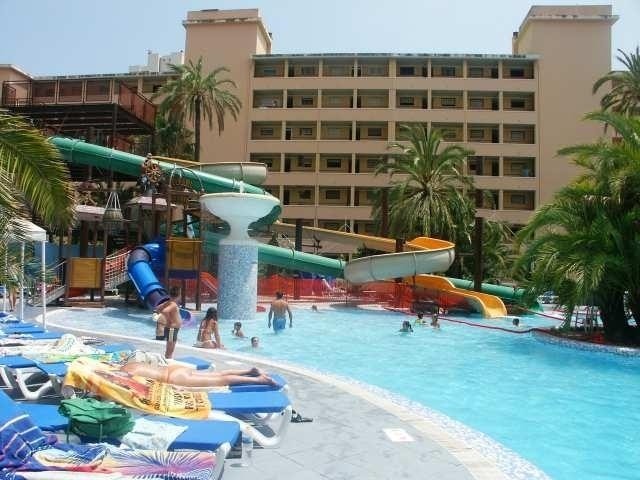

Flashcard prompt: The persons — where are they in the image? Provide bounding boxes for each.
[400,320,414,333]
[231,321,245,337]
[7,256,22,312]
[250,337,259,348]
[194,307,224,350]
[267,291,293,333]
[161,285,184,359]
[511,318,519,327]
[155,304,166,341]
[99,360,278,387]
[520,164,533,177]
[430,313,440,328]
[310,304,319,311]
[415,311,426,323]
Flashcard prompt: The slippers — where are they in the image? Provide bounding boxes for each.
[291,409,313,422]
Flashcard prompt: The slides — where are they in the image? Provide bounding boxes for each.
[187,206,509,319]
[125,226,340,321]
[1,159,270,249]
[43,135,544,315]
[197,270,266,314]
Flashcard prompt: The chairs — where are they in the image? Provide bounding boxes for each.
[1,314,66,340]
[0,344,292,480]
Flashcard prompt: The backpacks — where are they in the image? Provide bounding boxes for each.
[57,397,136,445]
